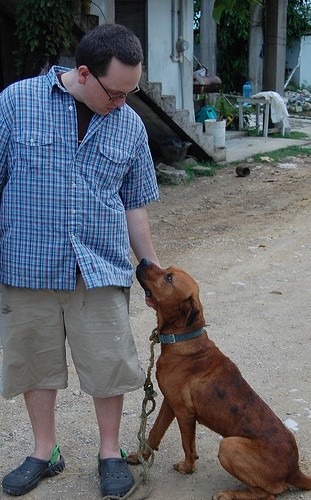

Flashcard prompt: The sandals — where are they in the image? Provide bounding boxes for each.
[98,447,134,498]
[3,442,64,496]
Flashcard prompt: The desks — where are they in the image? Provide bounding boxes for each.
[237,97,288,137]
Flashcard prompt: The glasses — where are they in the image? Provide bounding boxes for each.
[79,63,140,101]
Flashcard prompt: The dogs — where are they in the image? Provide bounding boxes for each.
[123,257,311,500]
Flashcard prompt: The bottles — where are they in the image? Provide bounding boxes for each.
[243,81,252,99]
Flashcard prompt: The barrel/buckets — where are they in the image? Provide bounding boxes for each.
[236,166,250,177]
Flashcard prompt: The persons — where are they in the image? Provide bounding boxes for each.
[0,22,163,500]
